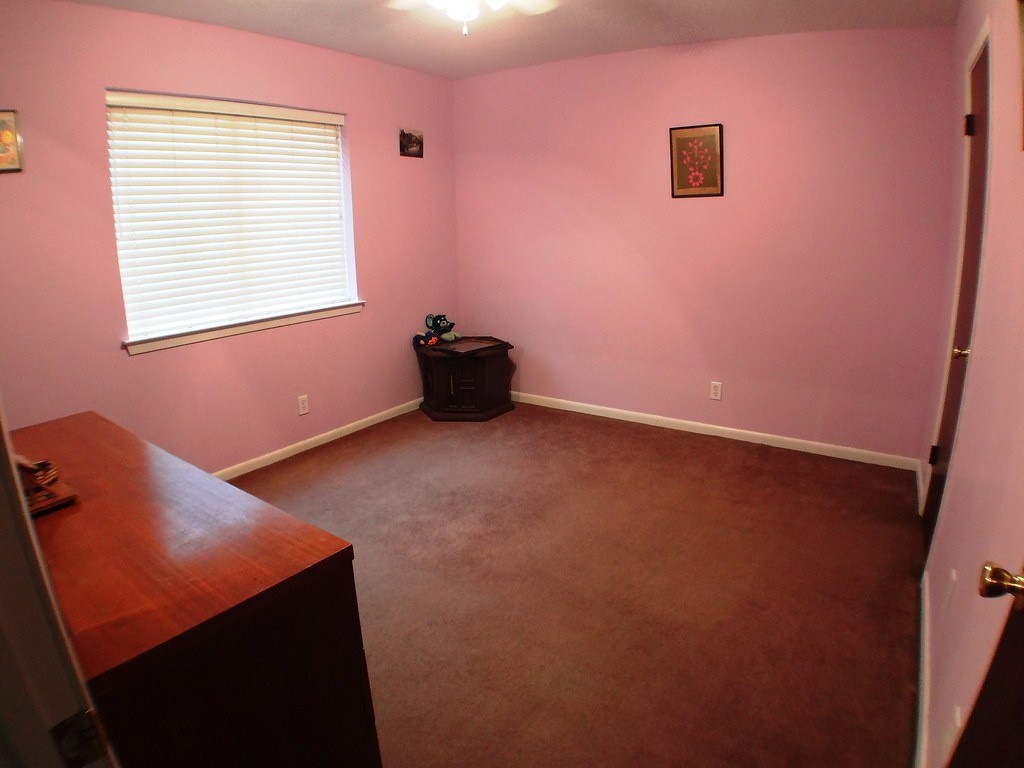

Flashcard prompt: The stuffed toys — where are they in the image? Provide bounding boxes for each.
[414,314,461,346]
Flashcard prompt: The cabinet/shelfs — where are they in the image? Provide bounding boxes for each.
[413,334,516,423]
[7,410,382,768]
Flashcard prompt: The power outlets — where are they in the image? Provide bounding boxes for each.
[710,381,722,400]
[298,395,309,415]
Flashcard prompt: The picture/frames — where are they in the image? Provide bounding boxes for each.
[669,123,724,199]
[0,110,22,172]
